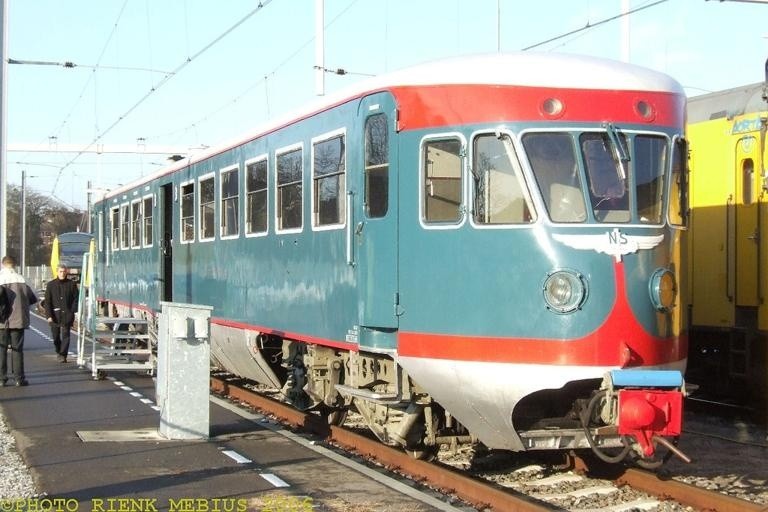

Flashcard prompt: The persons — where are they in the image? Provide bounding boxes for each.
[0,255,38,386]
[42,262,80,364]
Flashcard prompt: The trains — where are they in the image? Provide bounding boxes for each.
[50,231,97,296]
[666,80,767,418]
[83,49,692,477]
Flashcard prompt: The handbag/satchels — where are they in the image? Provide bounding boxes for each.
[0,286,8,324]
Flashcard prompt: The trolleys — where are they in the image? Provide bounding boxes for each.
[77,249,158,382]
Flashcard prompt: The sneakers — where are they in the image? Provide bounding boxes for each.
[15,380,28,386]
[60,355,66,363]
[57,353,62,363]
